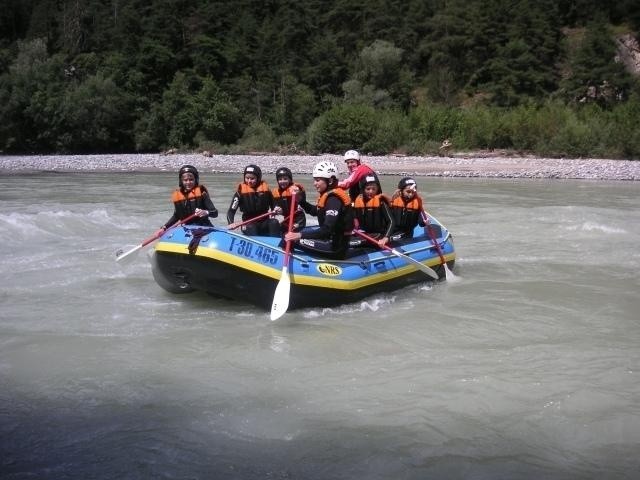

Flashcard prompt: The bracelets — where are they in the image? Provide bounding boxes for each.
[160,226,167,231]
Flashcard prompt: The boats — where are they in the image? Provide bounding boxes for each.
[149,211,456,309]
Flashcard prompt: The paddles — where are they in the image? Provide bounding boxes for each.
[116,213,199,266]
[421,207,455,281]
[271,189,297,322]
[352,228,439,279]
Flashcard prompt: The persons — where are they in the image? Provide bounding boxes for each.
[155,165,219,239]
[278,161,356,261]
[348,175,395,248]
[227,163,283,241]
[339,150,383,203]
[385,178,431,241]
[270,166,307,237]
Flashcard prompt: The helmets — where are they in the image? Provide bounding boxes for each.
[313,161,338,179]
[398,177,417,189]
[344,150,360,162]
[358,175,380,190]
[179,165,199,185]
[244,165,261,182]
[276,167,292,182]
